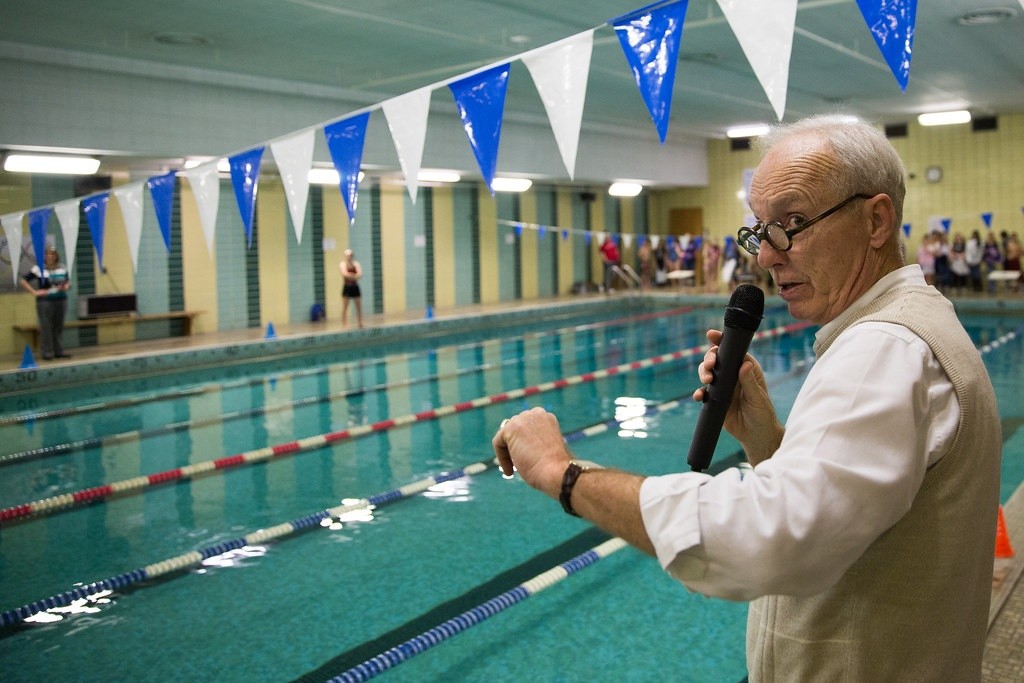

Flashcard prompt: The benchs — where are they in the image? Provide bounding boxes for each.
[13,310,210,352]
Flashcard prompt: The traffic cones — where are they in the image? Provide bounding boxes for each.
[19,344,37,369]
[261,323,276,338]
[425,306,434,319]
[993,504,1016,559]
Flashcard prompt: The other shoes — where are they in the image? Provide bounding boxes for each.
[57,351,72,358]
[42,353,54,361]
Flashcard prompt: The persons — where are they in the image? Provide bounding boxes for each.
[663,239,685,288]
[20,247,72,361]
[637,237,654,290]
[597,228,622,297]
[340,249,363,328]
[723,236,741,289]
[491,112,1004,682]
[918,230,1024,292]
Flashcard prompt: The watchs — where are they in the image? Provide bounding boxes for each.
[559,460,607,517]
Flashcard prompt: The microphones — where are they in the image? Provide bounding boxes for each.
[687,284,765,474]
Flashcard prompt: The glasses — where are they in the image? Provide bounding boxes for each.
[737,193,873,256]
